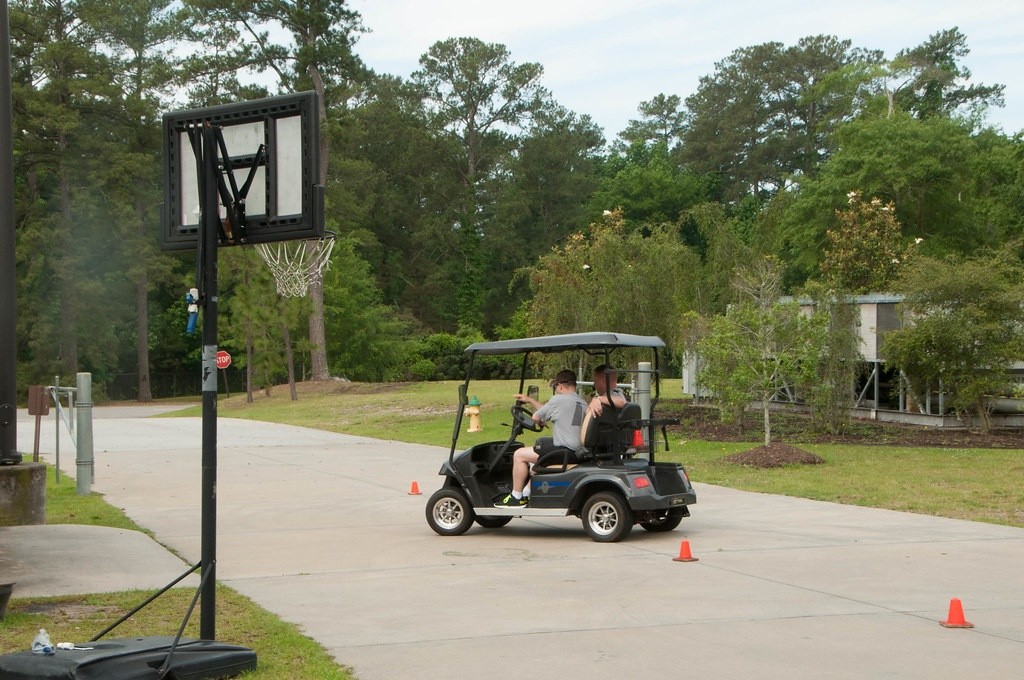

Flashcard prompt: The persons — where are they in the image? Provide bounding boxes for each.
[581,364,626,462]
[493,370,589,508]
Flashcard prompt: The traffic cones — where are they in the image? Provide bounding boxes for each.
[408,481,422,496]
[940,598,974,630]
[672,540,699,562]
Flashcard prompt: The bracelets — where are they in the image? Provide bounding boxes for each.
[593,394,601,400]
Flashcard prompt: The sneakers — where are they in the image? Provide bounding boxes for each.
[494,493,530,508]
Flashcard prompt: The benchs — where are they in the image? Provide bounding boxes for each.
[537,404,642,465]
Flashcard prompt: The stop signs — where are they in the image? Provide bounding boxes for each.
[216,351,231,368]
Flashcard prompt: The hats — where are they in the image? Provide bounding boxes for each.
[550,370,577,386]
